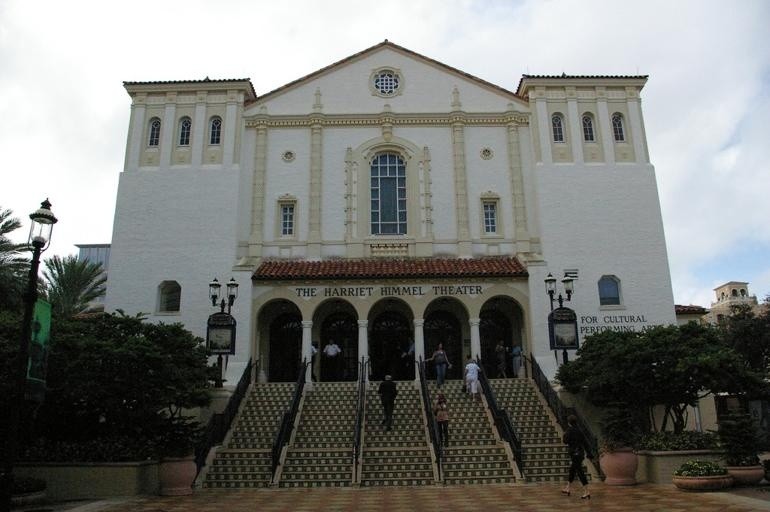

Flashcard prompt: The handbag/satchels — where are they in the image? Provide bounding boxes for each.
[462,384,466,392]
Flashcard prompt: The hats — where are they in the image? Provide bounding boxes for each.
[438,394,444,399]
[384,375,392,380]
[568,415,577,422]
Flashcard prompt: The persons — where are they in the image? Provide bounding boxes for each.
[495,339,508,378]
[404,337,415,380]
[423,342,451,388]
[433,393,451,448]
[312,342,318,382]
[464,354,482,402]
[560,415,594,500]
[377,375,398,430]
[322,338,341,382]
[511,340,524,377]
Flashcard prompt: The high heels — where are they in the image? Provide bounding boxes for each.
[581,494,590,499]
[561,489,570,495]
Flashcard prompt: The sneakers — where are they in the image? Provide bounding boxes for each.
[381,419,392,431]
[469,391,472,395]
[439,437,448,447]
[473,399,477,402]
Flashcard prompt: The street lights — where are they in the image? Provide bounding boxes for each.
[544,270,574,366]
[208,276,239,388]
[0,198,58,512]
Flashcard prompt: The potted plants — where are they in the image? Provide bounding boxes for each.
[672,408,770,492]
[594,402,642,486]
[155,431,198,496]
